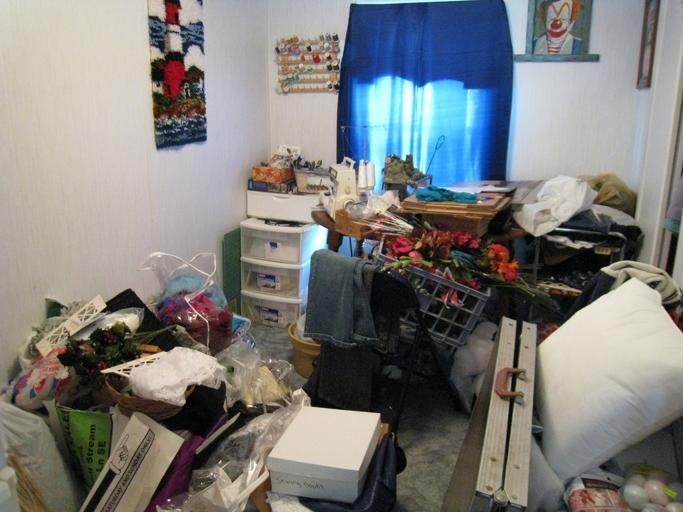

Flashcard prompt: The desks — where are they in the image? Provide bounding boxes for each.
[527,217,628,322]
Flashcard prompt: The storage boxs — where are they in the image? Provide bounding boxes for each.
[245,189,330,224]
[266,407,383,503]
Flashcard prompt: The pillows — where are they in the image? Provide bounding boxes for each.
[535,277,683,486]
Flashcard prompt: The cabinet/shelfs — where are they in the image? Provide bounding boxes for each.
[239,217,320,362]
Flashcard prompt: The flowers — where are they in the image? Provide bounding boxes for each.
[59,320,177,395]
[347,191,564,320]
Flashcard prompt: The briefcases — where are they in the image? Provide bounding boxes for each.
[438,316,539,510]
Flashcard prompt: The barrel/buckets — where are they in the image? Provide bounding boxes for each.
[286,321,321,379]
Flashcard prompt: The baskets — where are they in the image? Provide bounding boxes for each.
[105,371,196,421]
[374,232,491,360]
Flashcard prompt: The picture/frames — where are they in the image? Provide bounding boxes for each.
[635,0,660,89]
